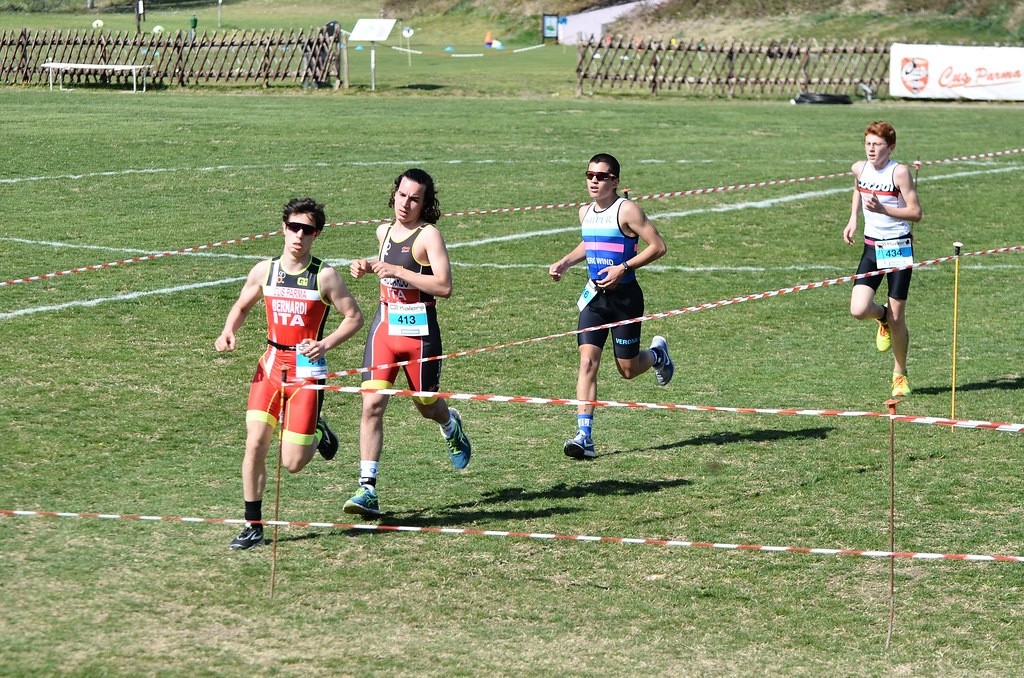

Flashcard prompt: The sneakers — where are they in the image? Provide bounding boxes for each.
[891,372,911,397]
[342,485,379,517]
[876,302,892,352]
[316,416,339,461]
[649,335,674,386]
[564,430,595,459]
[230,522,264,549]
[440,406,472,468]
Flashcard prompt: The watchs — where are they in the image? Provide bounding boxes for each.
[623,261,632,273]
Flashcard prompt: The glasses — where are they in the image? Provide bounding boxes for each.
[585,171,618,181]
[865,141,888,147]
[285,221,319,236]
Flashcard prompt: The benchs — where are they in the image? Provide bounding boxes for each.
[40,62,156,93]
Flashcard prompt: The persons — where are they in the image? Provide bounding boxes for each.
[214,198,364,550]
[842,121,922,397]
[549,153,673,459]
[342,168,471,517]
